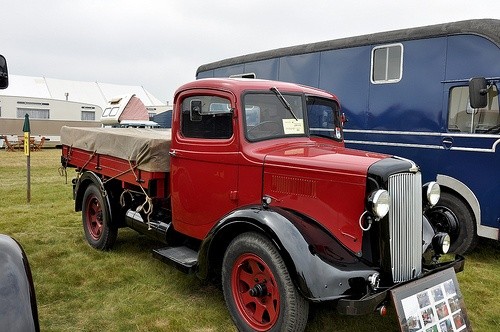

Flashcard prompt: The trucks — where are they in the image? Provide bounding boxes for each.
[158,16,500,254]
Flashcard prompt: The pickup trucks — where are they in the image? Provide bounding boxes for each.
[61,77,464,332]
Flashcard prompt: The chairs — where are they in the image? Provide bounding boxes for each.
[2,134,46,153]
[184,112,234,139]
